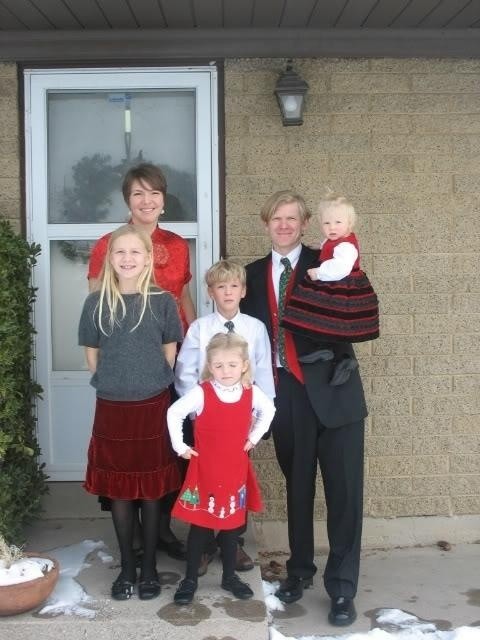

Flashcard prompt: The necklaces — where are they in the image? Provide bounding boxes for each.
[214,382,242,392]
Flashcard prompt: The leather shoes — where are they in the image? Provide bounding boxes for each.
[133,544,144,569]
[327,596,358,628]
[273,575,314,605]
[296,344,335,364]
[137,570,162,601]
[173,578,199,606]
[196,550,215,577]
[220,575,255,600]
[110,570,137,601]
[156,534,189,562]
[233,541,255,572]
[327,353,360,387]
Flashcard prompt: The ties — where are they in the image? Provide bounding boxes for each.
[277,256,295,375]
[224,321,235,334]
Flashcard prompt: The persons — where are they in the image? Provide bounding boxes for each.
[78,225,184,601]
[88,162,196,567]
[280,195,379,386]
[166,332,276,605]
[242,191,371,627]
[173,259,276,576]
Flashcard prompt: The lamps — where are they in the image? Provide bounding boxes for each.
[272,59,314,128]
